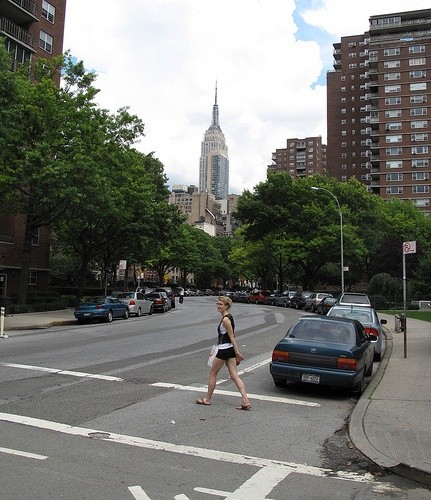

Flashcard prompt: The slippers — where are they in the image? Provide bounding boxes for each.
[236,404,252,410]
[195,398,211,405]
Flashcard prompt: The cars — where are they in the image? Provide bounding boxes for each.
[174,287,252,303]
[263,291,281,307]
[302,292,333,312]
[314,296,337,315]
[154,287,176,308]
[326,306,387,364]
[275,290,297,307]
[247,290,270,305]
[73,296,130,322]
[116,291,154,317]
[269,315,378,396]
[145,292,173,313]
[290,290,314,309]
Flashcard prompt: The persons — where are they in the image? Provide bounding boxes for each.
[196,297,252,410]
[179,286,184,303]
[123,286,129,292]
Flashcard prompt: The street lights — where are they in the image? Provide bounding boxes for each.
[310,185,344,292]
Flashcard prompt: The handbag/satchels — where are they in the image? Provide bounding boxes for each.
[207,345,218,368]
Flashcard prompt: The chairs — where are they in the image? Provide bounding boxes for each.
[301,327,351,343]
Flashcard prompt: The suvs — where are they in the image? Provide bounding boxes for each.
[334,292,371,308]
